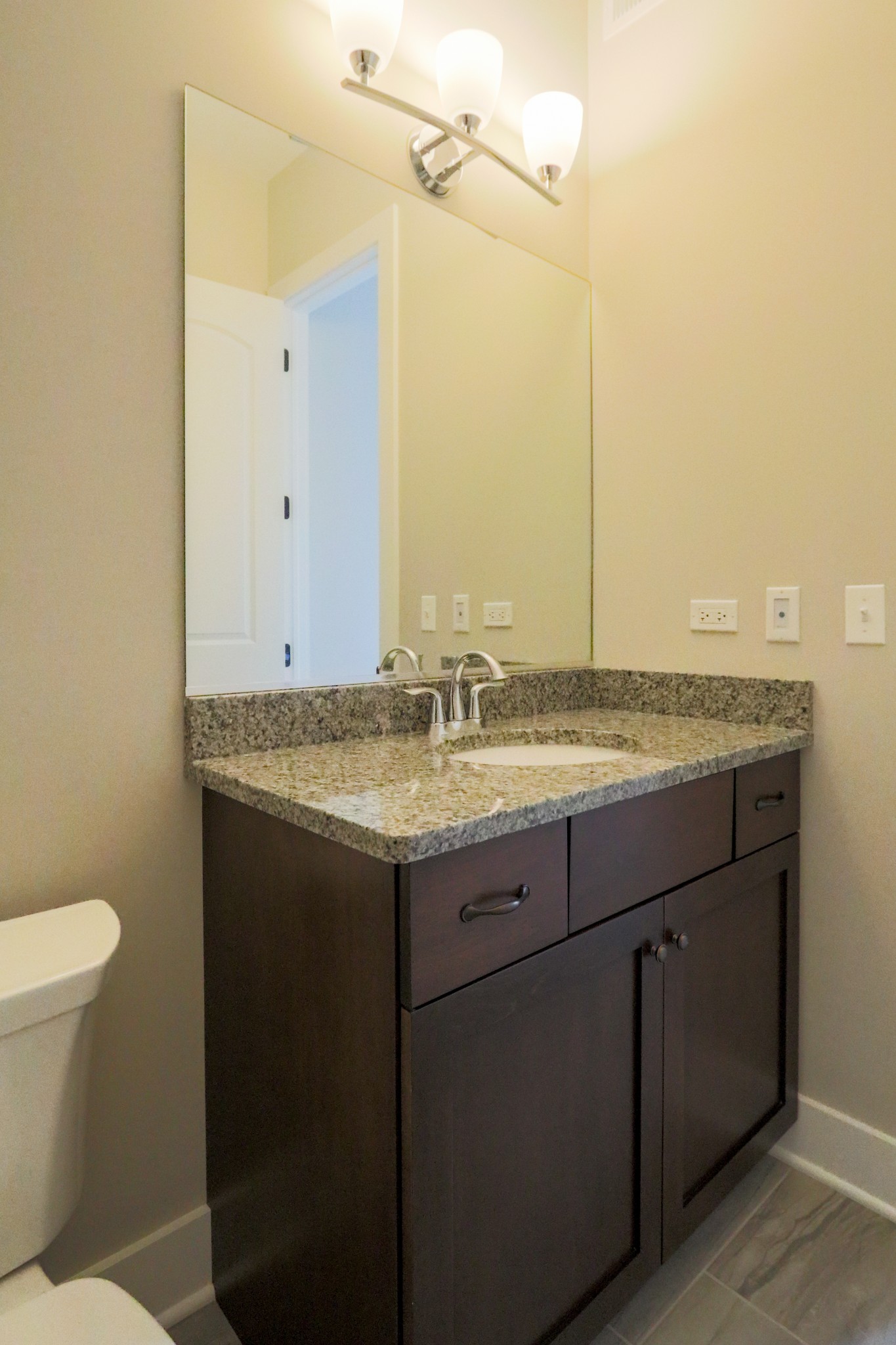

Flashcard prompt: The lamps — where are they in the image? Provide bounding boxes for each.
[331,4,582,206]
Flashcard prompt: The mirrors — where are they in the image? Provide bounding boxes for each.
[181,84,595,701]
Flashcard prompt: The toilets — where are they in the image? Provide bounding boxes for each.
[0,896,179,1345]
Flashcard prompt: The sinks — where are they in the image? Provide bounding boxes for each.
[435,727,645,768]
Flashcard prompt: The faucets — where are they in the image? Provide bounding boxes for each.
[448,651,509,723]
[379,647,422,674]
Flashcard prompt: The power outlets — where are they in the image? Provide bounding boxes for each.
[689,599,737,633]
[483,603,512,628]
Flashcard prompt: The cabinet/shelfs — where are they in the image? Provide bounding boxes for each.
[203,755,800,1345]
